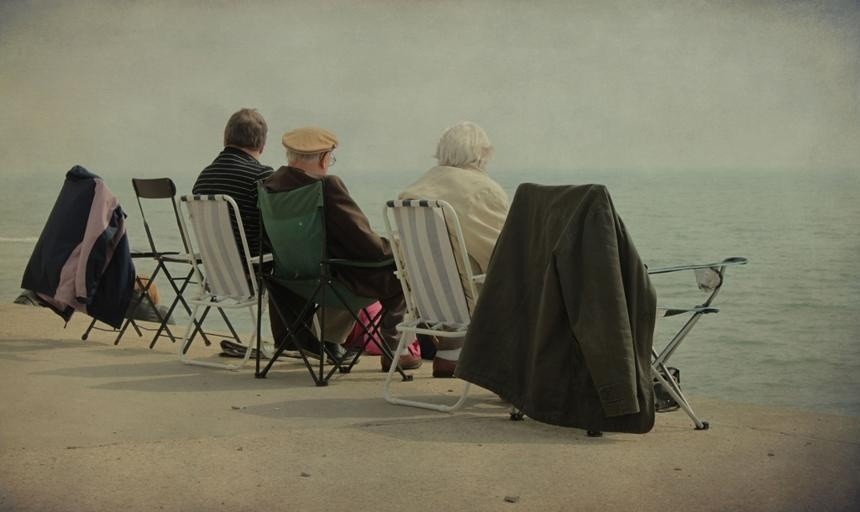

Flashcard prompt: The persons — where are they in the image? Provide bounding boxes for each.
[263,125,424,374]
[192,107,361,367]
[124,274,178,327]
[398,123,511,379]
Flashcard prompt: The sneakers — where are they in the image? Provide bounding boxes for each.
[274,337,308,358]
[301,338,322,360]
[381,354,423,371]
[325,343,361,364]
[433,357,457,377]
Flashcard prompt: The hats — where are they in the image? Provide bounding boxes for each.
[282,128,338,154]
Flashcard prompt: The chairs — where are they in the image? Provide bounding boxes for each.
[382,196,490,413]
[60,164,176,347]
[130,175,244,356]
[451,182,749,440]
[253,175,415,388]
[172,192,330,373]
